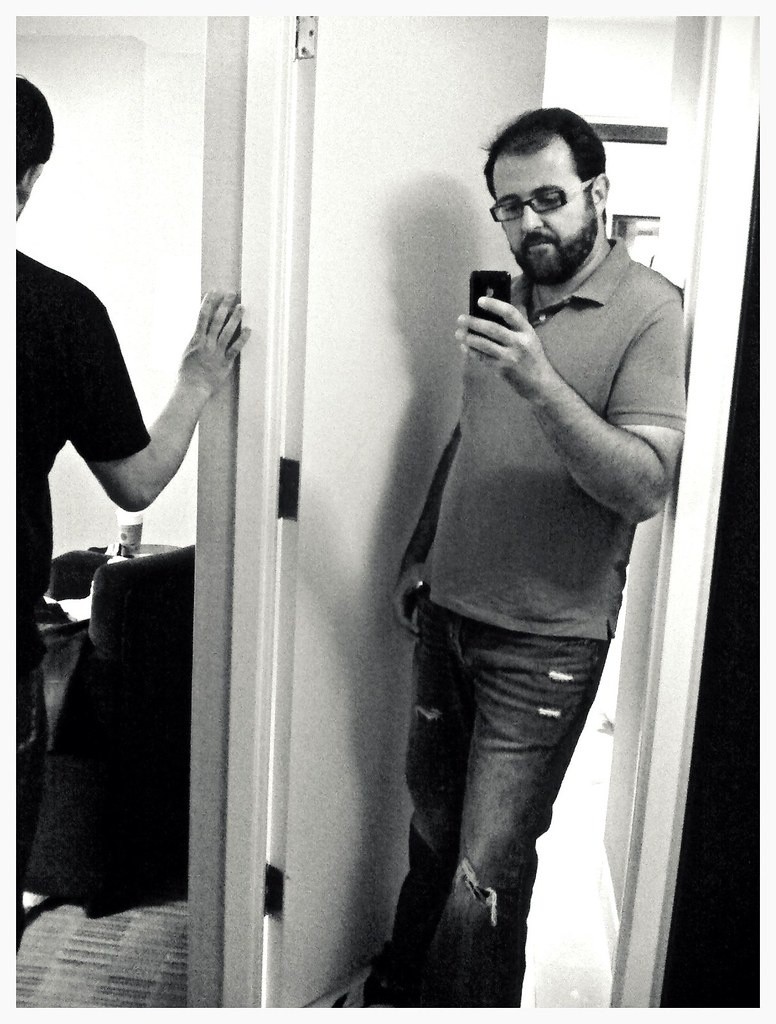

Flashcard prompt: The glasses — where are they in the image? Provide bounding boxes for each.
[490,177,596,222]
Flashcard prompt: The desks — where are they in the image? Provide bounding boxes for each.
[34,542,182,636]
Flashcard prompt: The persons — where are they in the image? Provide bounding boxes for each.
[363,106,685,1008]
[17,77,252,929]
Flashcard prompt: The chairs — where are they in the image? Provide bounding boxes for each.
[24,545,196,920]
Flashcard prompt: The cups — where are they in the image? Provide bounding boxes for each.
[116,519,143,553]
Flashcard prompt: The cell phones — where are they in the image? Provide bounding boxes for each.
[468,271,512,350]
